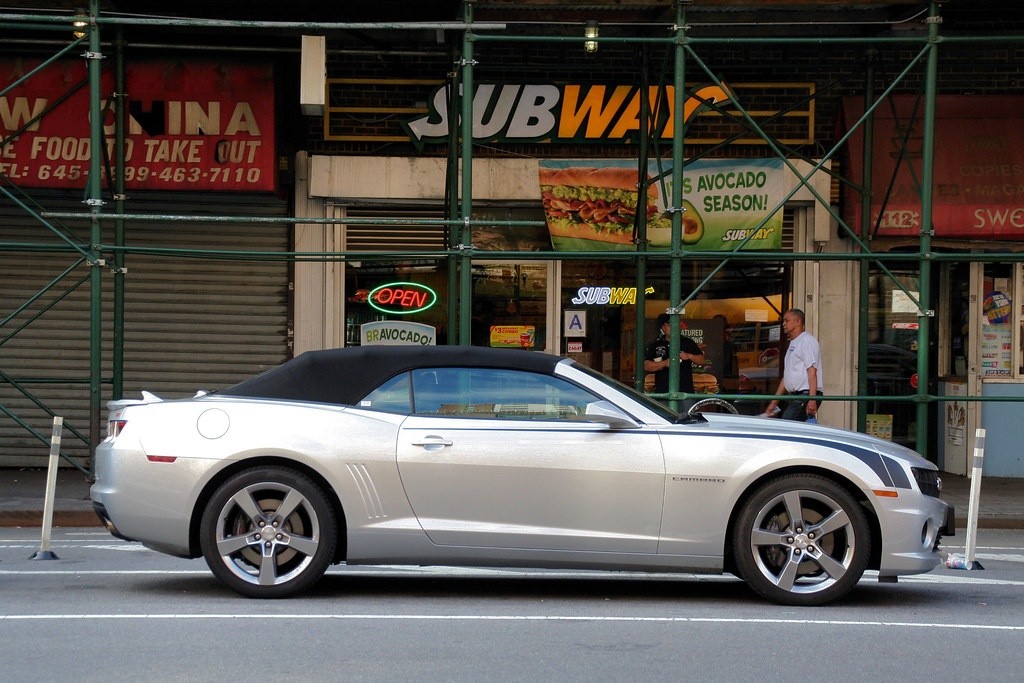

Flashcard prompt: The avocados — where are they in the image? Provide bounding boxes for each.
[681,199,705,244]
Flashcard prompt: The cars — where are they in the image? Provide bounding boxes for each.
[87,343,957,605]
[866,343,922,440]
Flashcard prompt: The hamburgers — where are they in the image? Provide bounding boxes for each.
[537,166,659,246]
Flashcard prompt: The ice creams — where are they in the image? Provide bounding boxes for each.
[646,215,684,248]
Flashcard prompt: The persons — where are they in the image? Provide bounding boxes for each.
[766,309,823,422]
[643,314,705,416]
